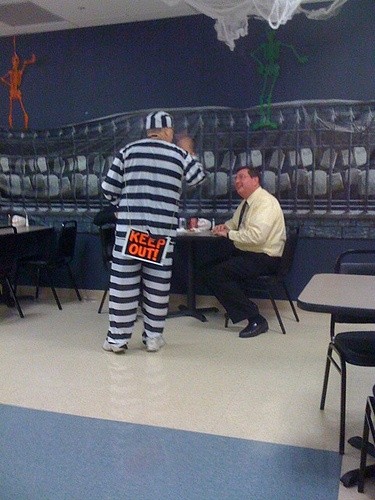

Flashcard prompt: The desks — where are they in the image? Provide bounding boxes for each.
[297,272,375,343]
[0,224,53,236]
[166,229,225,323]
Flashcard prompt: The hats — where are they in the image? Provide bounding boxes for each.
[145,111,175,130]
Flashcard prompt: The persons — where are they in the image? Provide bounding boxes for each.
[100,110,206,354]
[202,166,286,338]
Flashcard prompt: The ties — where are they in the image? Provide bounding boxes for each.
[237,201,247,232]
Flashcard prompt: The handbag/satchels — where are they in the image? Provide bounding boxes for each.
[122,228,171,266]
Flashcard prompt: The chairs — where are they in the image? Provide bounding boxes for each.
[316,250,375,493]
[0,205,118,318]
[226,227,300,334]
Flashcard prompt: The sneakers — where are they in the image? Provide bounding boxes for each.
[102,340,125,352]
[146,336,164,351]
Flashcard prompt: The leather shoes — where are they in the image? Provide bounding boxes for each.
[239,318,268,338]
